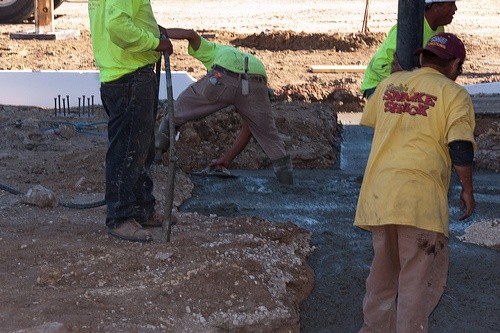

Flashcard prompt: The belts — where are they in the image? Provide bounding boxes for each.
[212,63,264,80]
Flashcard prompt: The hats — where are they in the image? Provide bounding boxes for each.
[413,32,466,63]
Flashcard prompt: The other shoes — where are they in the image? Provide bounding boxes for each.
[108,217,153,241]
[140,210,178,225]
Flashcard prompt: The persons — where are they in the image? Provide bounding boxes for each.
[352,33,477,333]
[88,0,178,242]
[153,27,294,185]
[362,0,457,99]
